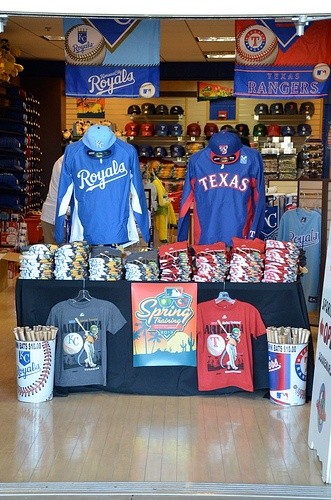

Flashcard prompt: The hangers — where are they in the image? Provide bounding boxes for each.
[297,189,319,216]
[215,281,235,305]
[70,279,92,304]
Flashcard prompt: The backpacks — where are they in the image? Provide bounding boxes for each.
[296,138,324,181]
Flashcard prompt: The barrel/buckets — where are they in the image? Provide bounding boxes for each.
[267,341,308,406]
[15,339,56,402]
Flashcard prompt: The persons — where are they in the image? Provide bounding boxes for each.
[54,124,150,248]
[41,153,66,245]
[180,132,264,246]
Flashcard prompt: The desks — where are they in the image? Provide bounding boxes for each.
[15,277,315,403]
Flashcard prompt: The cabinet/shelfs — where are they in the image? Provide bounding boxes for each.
[130,114,183,141]
[253,114,310,142]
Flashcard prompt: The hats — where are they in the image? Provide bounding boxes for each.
[80,125,117,159]
[204,101,315,139]
[0,136,27,211]
[208,131,243,165]
[119,103,202,158]
